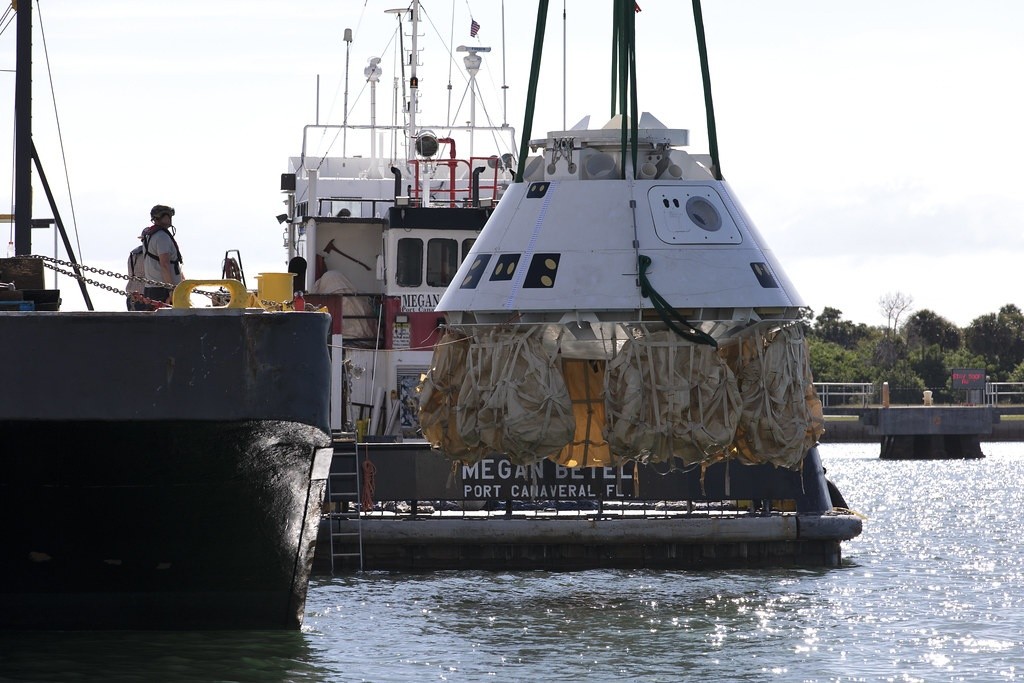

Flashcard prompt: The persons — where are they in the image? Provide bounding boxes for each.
[126,227,153,311]
[142,204,188,313]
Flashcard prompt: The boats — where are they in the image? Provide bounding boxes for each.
[277,0,862,565]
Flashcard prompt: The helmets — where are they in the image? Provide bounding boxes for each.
[141,227,152,242]
[150,205,175,220]
